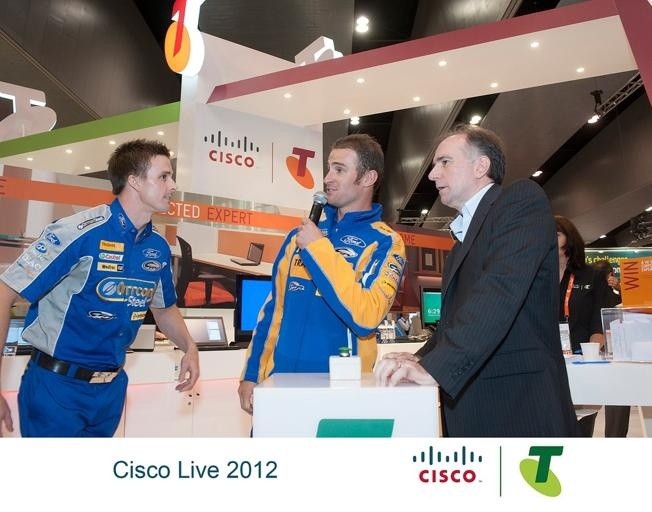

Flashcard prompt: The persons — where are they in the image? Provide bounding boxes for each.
[368,122,584,437]
[550,212,611,437]
[593,258,630,436]
[1,137,202,438]
[235,131,409,438]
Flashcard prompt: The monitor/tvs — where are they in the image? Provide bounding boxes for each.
[235,273,272,342]
[420,284,442,329]
[5,316,35,355]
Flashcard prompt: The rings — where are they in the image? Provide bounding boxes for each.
[395,356,403,368]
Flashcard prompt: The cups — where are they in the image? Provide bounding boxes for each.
[580,342,600,363]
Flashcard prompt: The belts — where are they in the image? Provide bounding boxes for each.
[30,347,124,384]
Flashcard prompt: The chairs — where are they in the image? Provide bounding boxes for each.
[175,233,238,308]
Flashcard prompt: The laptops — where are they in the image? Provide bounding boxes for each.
[182,317,240,350]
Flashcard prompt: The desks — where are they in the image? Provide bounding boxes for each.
[252,373,440,438]
[170,254,275,307]
[564,352,652,407]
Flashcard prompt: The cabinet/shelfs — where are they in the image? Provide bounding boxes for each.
[0,342,256,437]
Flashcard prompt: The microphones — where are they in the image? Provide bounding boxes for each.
[309,191,329,226]
